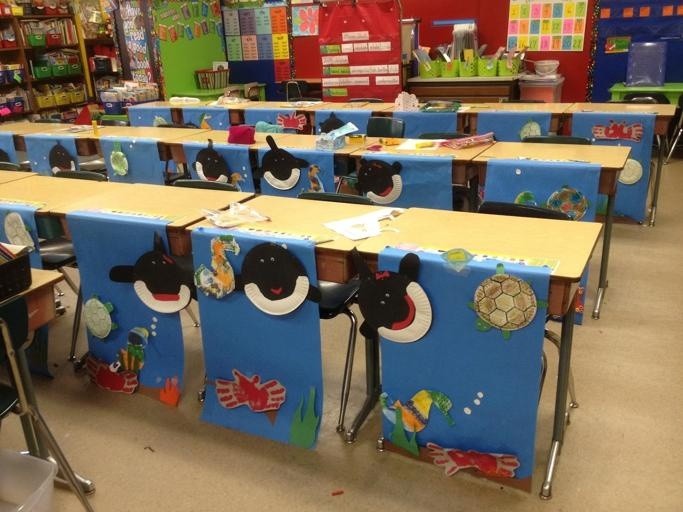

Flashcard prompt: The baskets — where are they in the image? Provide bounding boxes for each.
[418,55,520,78]
[195,68,230,89]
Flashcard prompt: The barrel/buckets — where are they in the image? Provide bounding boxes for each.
[534,60,560,75]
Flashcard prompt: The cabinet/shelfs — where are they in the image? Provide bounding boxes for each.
[0,0,94,117]
